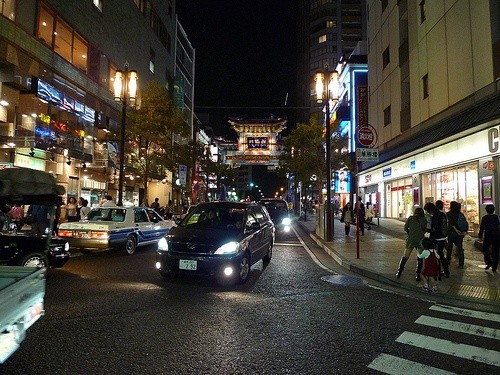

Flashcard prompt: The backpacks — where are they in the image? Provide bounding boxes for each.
[455,213,468,232]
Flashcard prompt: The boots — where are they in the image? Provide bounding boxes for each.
[396,256,408,278]
[345,226,350,236]
[416,258,423,281]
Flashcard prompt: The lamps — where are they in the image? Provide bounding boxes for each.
[50,152,55,162]
[82,161,86,168]
[29,147,35,157]
[66,157,72,165]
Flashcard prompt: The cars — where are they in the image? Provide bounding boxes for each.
[155,201,277,284]
[186,205,204,224]
[0,230,71,271]
[58,206,178,255]
[260,198,291,228]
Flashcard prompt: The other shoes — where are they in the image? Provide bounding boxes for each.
[485,263,493,270]
[431,286,438,294]
[368,228,371,230]
[492,265,497,272]
[422,283,429,292]
[457,265,463,269]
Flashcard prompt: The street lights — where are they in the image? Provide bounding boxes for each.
[313,69,339,242]
[112,64,138,205]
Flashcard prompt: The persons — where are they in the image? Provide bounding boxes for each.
[395,208,427,280]
[335,200,374,237]
[478,204,500,271]
[416,237,440,293]
[289,199,327,217]
[424,200,469,281]
[0,195,215,238]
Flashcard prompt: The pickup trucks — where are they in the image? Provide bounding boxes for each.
[0,266,47,364]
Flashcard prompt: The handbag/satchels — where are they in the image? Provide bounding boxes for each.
[341,216,344,223]
[366,218,370,223]
[453,226,466,234]
